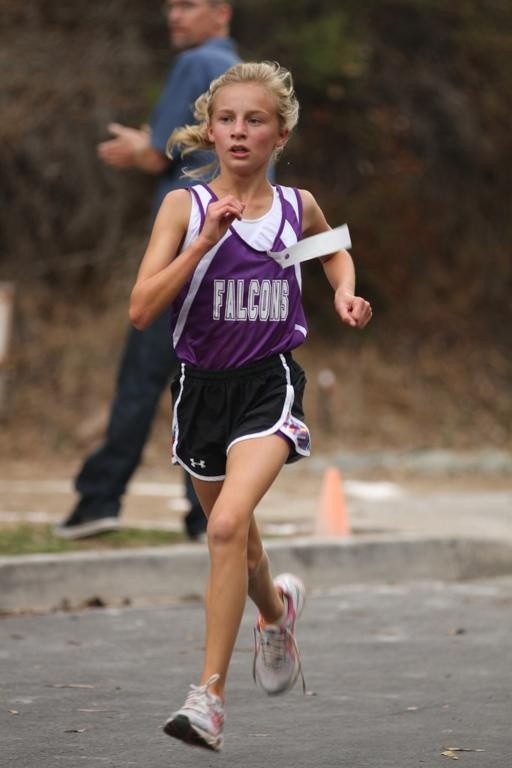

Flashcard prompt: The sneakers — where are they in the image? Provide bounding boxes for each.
[256,574,306,694]
[164,673,224,751]
[54,498,119,539]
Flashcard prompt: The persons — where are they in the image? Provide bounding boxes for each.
[128,61,372,753]
[53,0,275,542]
